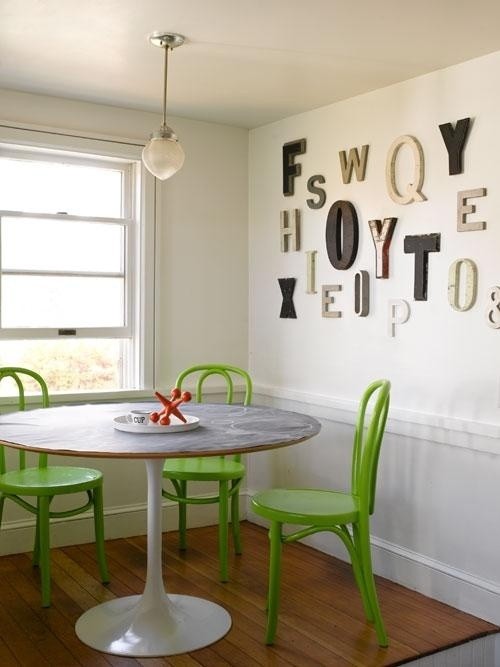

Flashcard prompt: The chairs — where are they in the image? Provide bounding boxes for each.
[247,375,396,652]
[0,361,111,607]
[153,360,255,588]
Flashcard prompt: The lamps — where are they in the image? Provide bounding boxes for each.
[135,29,190,183]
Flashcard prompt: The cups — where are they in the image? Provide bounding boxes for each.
[125,408,152,426]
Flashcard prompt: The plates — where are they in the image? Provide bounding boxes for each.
[114,415,200,434]
